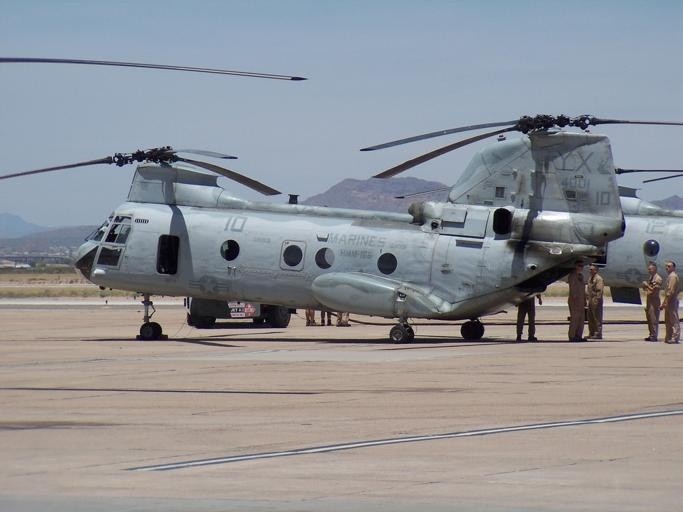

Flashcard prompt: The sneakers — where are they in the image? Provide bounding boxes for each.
[568,333,603,341]
[645,335,656,341]
[527,335,537,341]
[515,334,521,342]
[665,337,679,344]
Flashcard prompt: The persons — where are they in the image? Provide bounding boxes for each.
[515,293,542,342]
[659,260,681,344]
[584,264,604,338]
[568,260,587,342]
[305,307,351,327]
[641,261,662,341]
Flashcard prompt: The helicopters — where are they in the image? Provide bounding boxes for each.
[0,55,307,85]
[393,166,683,314]
[0,109,683,341]
